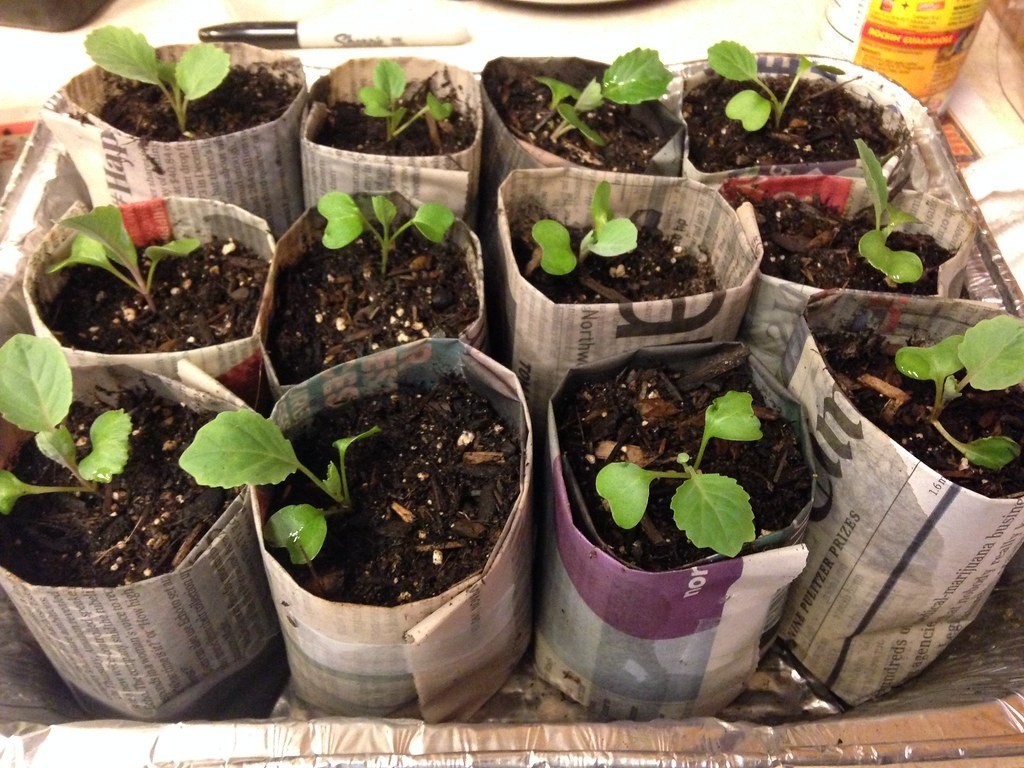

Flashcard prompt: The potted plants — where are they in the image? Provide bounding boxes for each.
[0,22,1024,718]
